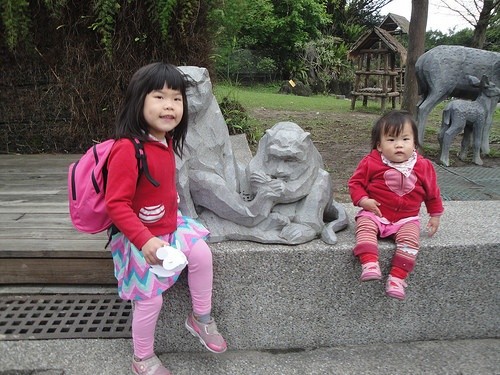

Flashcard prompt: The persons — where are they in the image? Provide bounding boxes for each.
[347,109,445,300]
[104,62,228,375]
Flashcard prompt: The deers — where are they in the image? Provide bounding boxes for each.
[412,42,500,167]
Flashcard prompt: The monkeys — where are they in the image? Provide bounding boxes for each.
[176,65,281,227]
[246,121,349,244]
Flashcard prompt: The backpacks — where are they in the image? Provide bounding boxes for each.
[67,135,160,249]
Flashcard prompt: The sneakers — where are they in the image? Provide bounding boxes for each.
[385,274,409,300]
[184,311,229,355]
[360,262,383,281]
[131,354,170,375]
